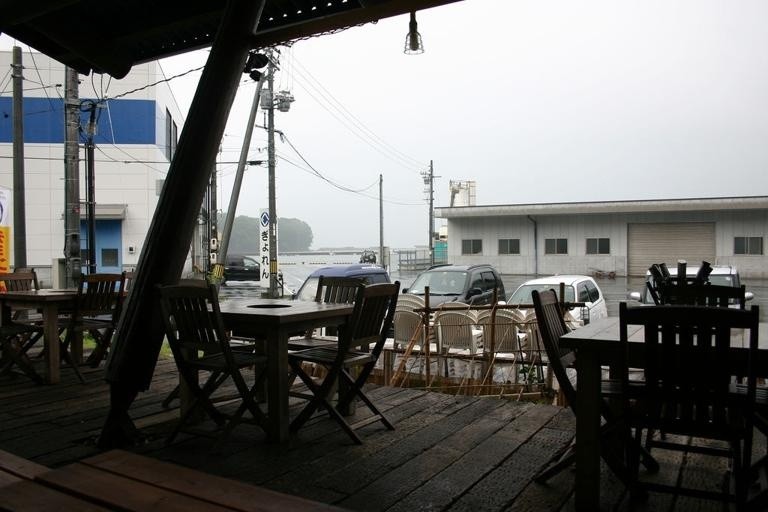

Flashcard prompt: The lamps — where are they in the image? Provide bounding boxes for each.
[400,11,424,54]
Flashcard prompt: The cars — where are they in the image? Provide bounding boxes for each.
[221,255,282,285]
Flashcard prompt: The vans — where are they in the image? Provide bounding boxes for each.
[628,264,754,334]
[496,274,609,345]
[294,264,392,308]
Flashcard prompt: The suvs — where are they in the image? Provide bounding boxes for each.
[359,250,377,263]
[383,263,506,345]
